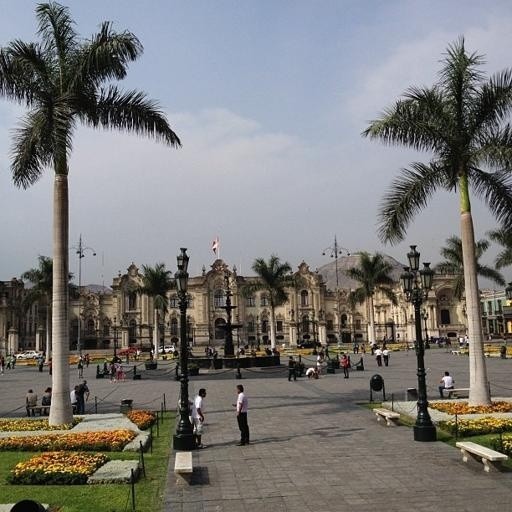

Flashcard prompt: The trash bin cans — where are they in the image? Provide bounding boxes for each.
[371,374,383,392]
[120,400,133,414]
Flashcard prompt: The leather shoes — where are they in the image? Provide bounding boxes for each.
[236,440,250,446]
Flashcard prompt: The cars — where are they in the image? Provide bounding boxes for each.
[16,350,42,359]
[151,345,176,354]
[297,341,322,349]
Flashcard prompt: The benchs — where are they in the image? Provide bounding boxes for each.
[174,450,194,488]
[444,388,470,399]
[28,404,77,415]
[484,353,489,358]
[373,408,401,427]
[452,350,461,355]
[456,441,509,474]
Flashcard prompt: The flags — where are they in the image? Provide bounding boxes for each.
[212,236,219,255]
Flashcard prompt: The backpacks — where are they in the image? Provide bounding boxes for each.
[76,384,83,395]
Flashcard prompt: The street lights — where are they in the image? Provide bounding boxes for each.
[421,308,430,349]
[173,247,196,451]
[399,245,436,441]
[76,234,96,356]
[107,317,123,355]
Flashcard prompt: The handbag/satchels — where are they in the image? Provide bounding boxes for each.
[43,396,46,406]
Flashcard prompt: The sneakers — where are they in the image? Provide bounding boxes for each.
[193,443,207,448]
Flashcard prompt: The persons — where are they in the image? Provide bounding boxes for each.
[47,357,52,376]
[137,347,141,360]
[368,342,377,355]
[77,380,90,415]
[191,387,207,449]
[11,354,16,370]
[340,354,350,379]
[436,334,469,347]
[317,353,328,373]
[354,343,359,353]
[42,387,52,414]
[383,347,390,366]
[362,343,366,354]
[438,371,455,398]
[319,346,325,360]
[305,367,319,379]
[324,345,330,360]
[5,355,11,370]
[77,353,123,381]
[70,385,79,415]
[287,355,298,382]
[37,356,45,371]
[25,388,38,417]
[0,356,6,376]
[374,346,382,366]
[232,385,249,446]
[313,346,316,355]
